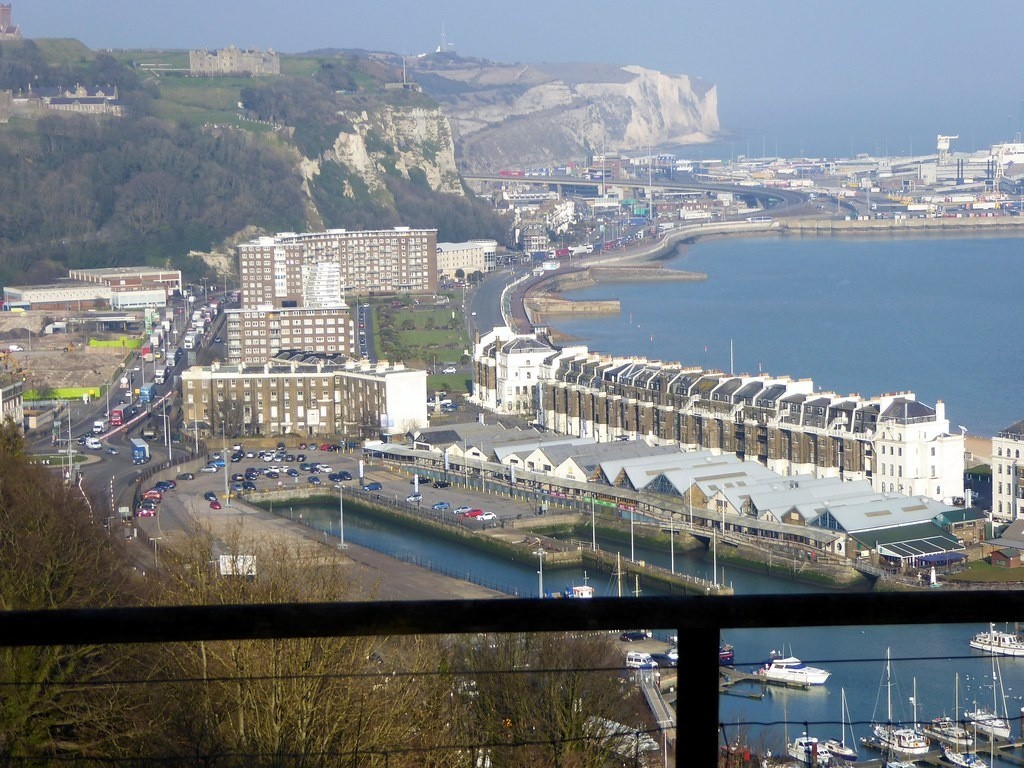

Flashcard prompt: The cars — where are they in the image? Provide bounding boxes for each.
[475,510,496,521]
[443,366,456,374]
[464,508,483,517]
[77,277,451,503]
[0,348,13,354]
[453,506,472,514]
[432,502,451,510]
[425,391,460,412]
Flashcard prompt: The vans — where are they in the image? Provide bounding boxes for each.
[626,652,659,671]
[9,345,23,352]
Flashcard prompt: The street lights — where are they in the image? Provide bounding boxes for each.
[154,396,167,446]
[177,306,184,334]
[123,367,139,405]
[656,720,673,768]
[158,414,172,462]
[60,398,80,477]
[181,298,187,324]
[103,383,113,421]
[56,438,81,480]
[202,278,209,303]
[189,283,195,311]
[184,401,199,453]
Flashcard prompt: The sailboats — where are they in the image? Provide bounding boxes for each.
[542,551,1024,768]
